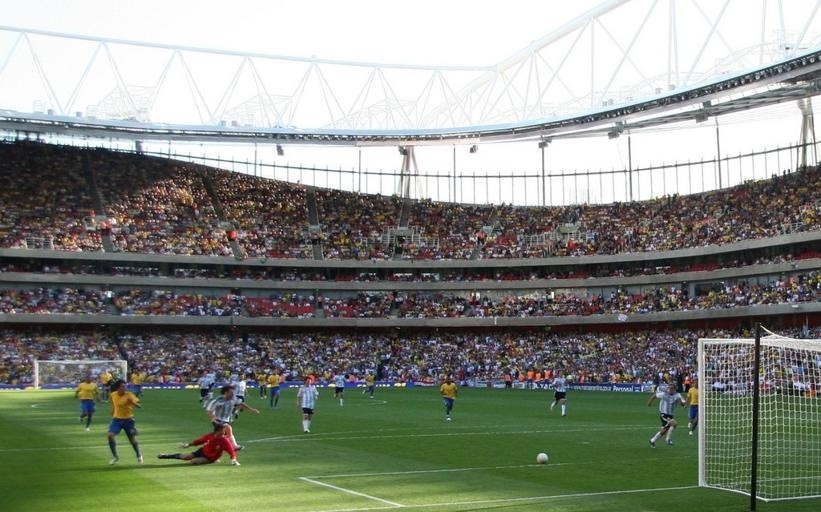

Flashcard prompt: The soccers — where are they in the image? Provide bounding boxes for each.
[537,452,548,464]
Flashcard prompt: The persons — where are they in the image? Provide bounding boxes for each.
[550,369,569,417]
[648,383,685,450]
[439,376,460,420]
[155,420,241,468]
[106,380,144,466]
[74,375,102,432]
[684,376,700,435]
[207,386,261,453]
[0,139,821,408]
[296,376,322,435]
